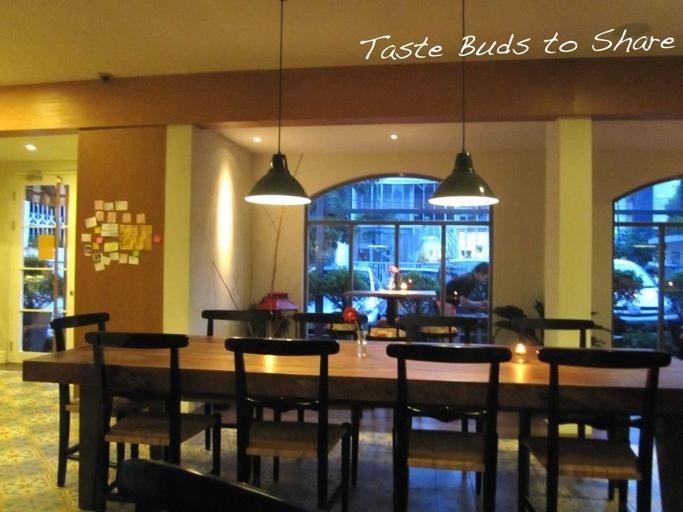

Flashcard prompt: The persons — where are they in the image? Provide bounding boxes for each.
[435,262,489,344]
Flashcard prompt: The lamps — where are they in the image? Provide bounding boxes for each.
[428,0,501,207]
[243,0,312,207]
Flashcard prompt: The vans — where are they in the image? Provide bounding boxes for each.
[613,258,679,324]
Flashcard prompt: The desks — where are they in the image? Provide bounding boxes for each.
[344,290,437,324]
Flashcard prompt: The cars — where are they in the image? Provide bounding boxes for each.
[308,265,385,322]
[388,267,458,291]
